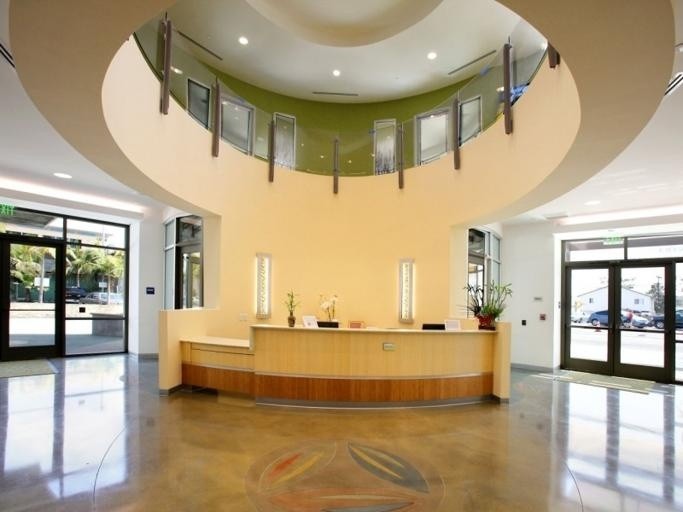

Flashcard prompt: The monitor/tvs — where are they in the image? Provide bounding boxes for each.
[421,322,445,331]
[317,321,337,326]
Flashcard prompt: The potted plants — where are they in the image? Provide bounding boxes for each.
[281,288,302,327]
[465,281,513,330]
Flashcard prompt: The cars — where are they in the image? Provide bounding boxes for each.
[654,309,683,329]
[589,309,649,328]
[79,292,126,304]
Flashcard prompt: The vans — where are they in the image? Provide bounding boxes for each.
[65,287,91,302]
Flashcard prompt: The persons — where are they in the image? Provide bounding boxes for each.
[622,310,632,329]
